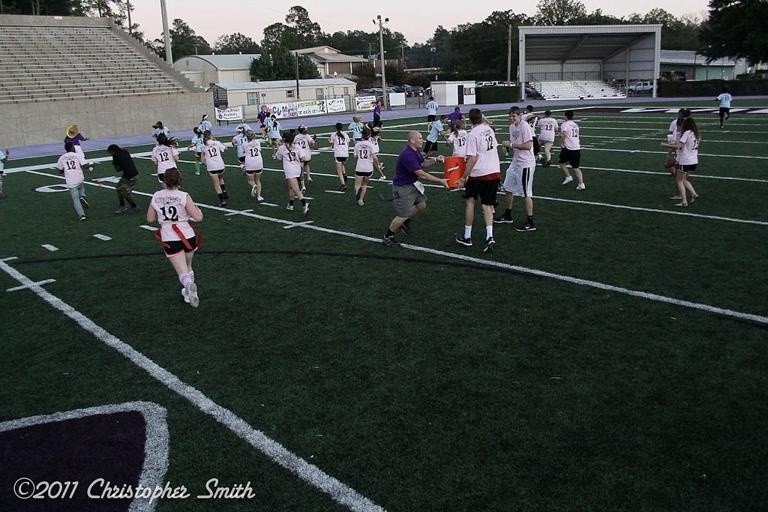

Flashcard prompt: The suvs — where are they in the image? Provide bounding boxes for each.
[629,81,656,95]
[662,70,687,80]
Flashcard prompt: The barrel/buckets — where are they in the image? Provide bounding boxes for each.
[443,156,467,190]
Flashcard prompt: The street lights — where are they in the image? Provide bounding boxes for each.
[375,50,388,60]
[371,13,391,110]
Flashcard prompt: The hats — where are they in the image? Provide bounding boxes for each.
[66,124,80,138]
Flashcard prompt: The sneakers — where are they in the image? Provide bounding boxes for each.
[250,184,264,202]
[302,203,310,217]
[356,186,365,206]
[563,176,573,186]
[217,197,229,207]
[482,237,495,254]
[493,216,515,225]
[536,156,552,166]
[286,203,295,211]
[577,182,585,190]
[188,281,200,309]
[80,195,88,207]
[515,223,537,232]
[372,162,387,180]
[669,191,699,207]
[381,236,399,247]
[400,222,413,236]
[340,173,348,191]
[79,216,86,222]
[455,235,473,246]
[182,287,190,302]
[115,206,140,214]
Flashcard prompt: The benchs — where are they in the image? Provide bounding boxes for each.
[528,80,626,100]
[0,28,193,104]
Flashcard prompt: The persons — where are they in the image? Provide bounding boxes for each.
[714,85,733,129]
[660,107,700,210]
[58,97,586,254]
[0,148,9,197]
[147,168,203,308]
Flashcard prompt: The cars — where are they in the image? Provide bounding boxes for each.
[368,85,435,98]
[478,79,538,97]
